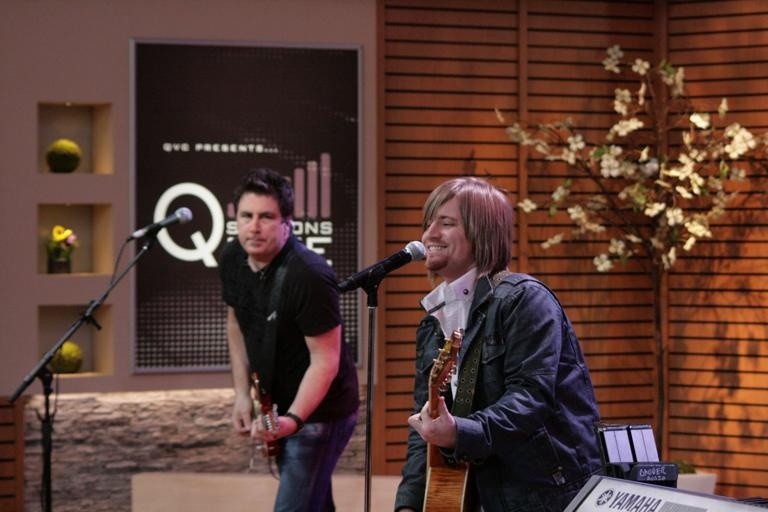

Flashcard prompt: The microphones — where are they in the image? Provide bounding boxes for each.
[126,207,194,242]
[337,241,427,295]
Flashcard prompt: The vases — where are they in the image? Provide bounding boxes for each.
[676,469,717,496]
[48,256,70,275]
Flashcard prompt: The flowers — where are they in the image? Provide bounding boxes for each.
[491,41,767,474]
[48,225,79,258]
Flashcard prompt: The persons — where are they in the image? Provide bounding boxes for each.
[386,175,603,509]
[215,165,362,512]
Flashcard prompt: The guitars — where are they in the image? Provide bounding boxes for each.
[423,330,475,511]
[247,372,281,457]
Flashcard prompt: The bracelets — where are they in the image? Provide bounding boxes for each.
[284,412,307,434]
